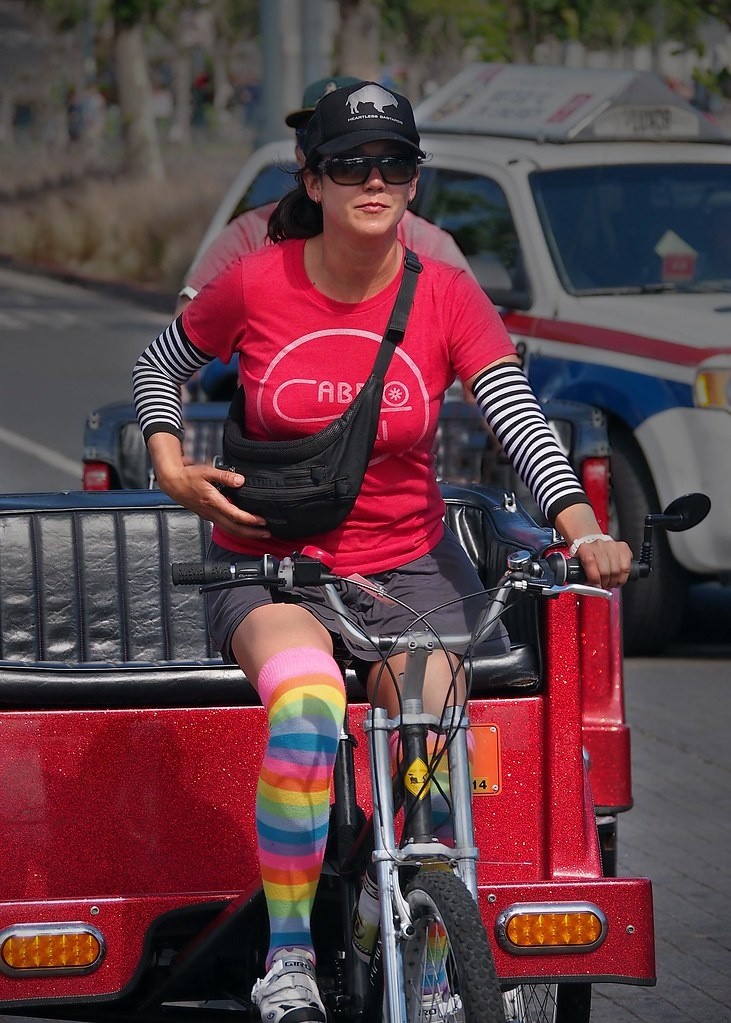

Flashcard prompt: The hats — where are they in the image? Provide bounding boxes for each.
[304,81,426,160]
[286,76,362,128]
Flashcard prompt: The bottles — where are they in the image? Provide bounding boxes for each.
[348,857,399,963]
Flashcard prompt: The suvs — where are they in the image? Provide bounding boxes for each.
[166,59,731,663]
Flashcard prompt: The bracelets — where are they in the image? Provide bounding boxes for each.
[569,534,614,557]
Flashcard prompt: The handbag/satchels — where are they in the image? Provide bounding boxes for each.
[217,245,423,535]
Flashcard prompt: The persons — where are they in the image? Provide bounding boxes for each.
[175,75,502,456]
[131,83,634,1023]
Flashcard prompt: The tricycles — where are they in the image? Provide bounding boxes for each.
[0,389,660,1023]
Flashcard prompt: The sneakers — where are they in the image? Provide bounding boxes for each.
[407,990,462,1023]
[251,946,328,1023]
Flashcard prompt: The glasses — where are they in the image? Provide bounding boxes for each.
[295,130,310,156]
[316,154,421,186]
